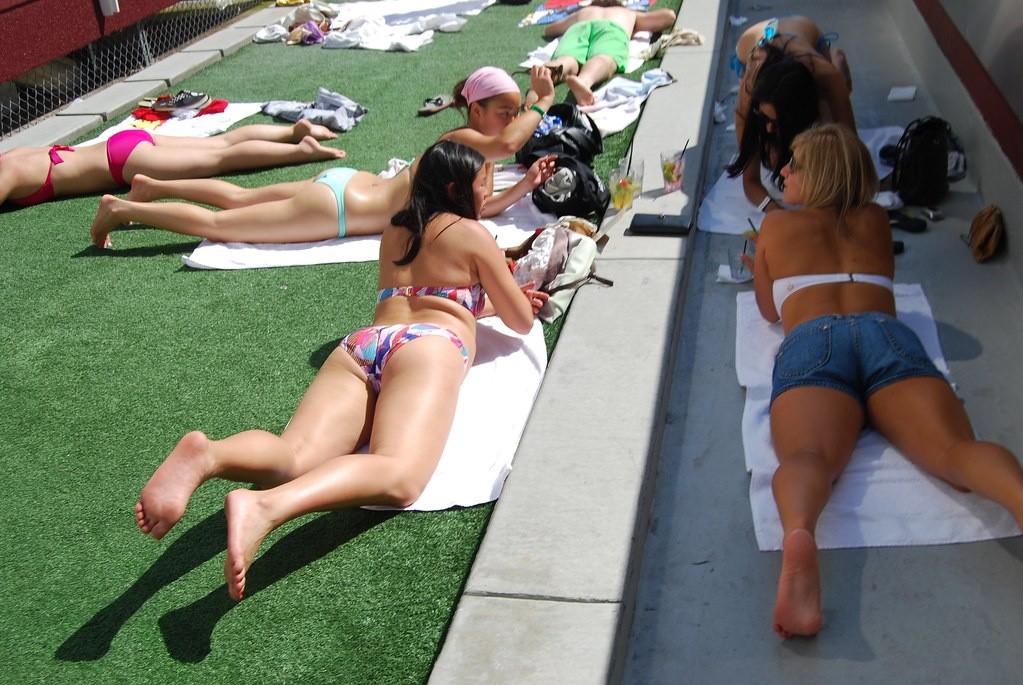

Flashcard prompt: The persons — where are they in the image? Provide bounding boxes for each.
[524,0,676,110]
[135,139,549,600]
[0,119,346,209]
[740,125,1023,640]
[92,66,557,248]
[725,16,857,215]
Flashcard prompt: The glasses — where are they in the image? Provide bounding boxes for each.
[790,156,803,171]
[759,111,778,125]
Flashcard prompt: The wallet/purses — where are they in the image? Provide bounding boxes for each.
[629,214,695,235]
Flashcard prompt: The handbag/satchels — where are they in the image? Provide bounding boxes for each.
[960,204,1004,263]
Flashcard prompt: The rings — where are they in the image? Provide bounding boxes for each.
[532,298,535,304]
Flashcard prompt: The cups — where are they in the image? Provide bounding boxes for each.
[660,148,686,192]
[609,167,634,209]
[619,158,645,198]
[744,229,761,254]
[727,246,753,281]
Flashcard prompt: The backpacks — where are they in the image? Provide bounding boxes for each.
[893,116,951,205]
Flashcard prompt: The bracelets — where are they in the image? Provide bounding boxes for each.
[760,196,773,211]
[530,105,545,119]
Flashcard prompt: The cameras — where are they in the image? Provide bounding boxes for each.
[545,65,563,82]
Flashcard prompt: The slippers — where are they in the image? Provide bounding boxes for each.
[888,209,927,232]
[150,89,213,111]
[418,94,454,116]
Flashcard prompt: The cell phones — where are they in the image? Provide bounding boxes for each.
[921,207,945,222]
